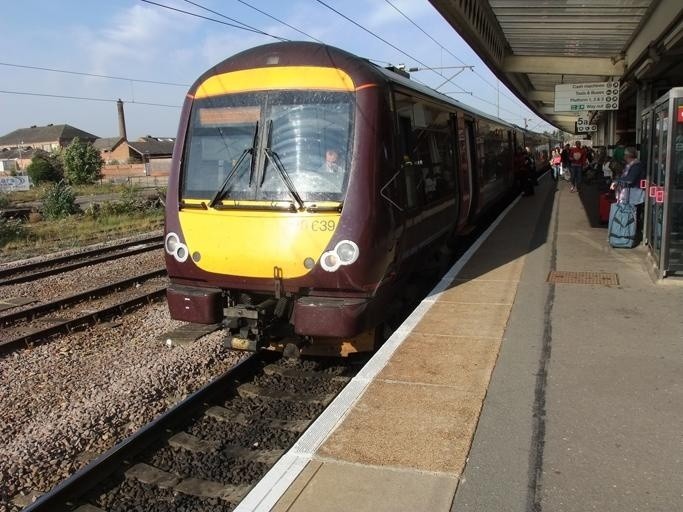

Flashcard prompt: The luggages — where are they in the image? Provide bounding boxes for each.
[608,180,637,249]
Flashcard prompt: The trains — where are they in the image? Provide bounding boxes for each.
[161,42,564,361]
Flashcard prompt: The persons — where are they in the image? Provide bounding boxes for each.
[316,147,345,174]
[515,139,645,241]
